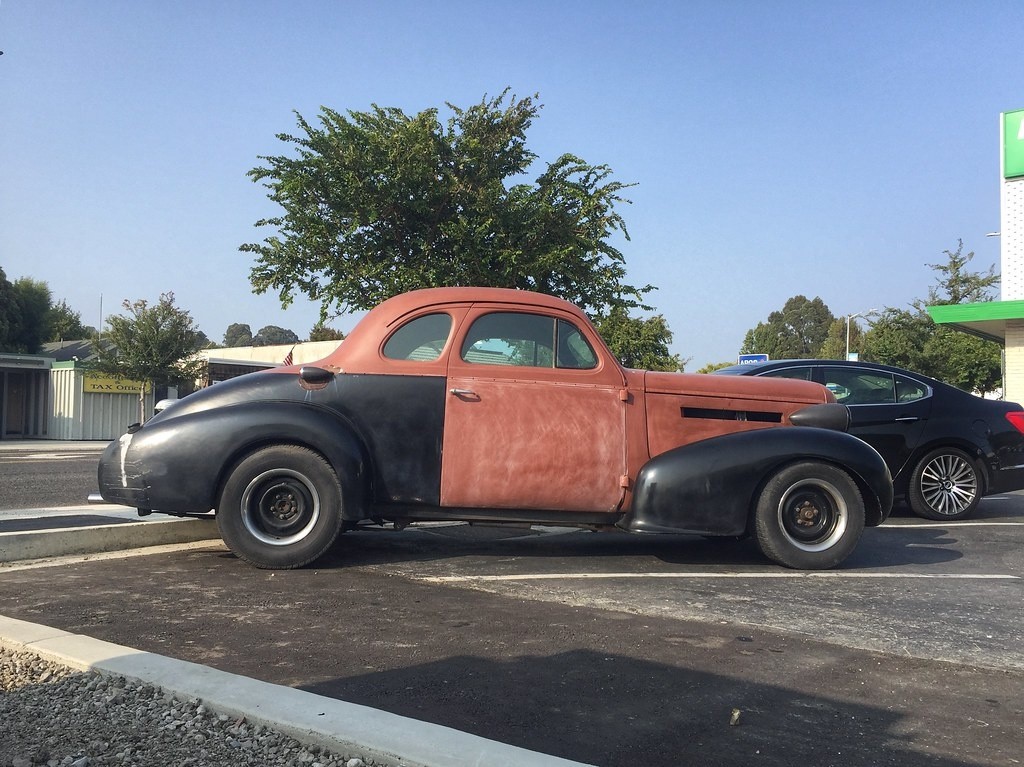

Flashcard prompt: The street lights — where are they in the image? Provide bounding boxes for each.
[845,308,878,362]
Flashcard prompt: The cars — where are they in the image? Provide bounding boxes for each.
[98,288,897,572]
[702,357,1024,522]
